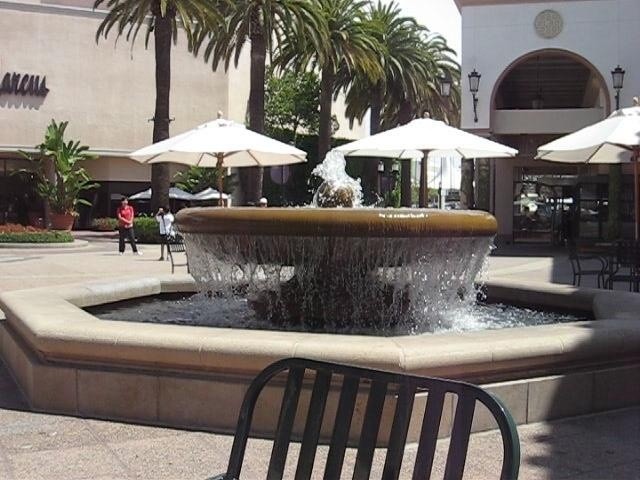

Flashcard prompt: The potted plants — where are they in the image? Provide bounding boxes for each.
[9,118,102,230]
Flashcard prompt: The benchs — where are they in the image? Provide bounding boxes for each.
[566,232,640,293]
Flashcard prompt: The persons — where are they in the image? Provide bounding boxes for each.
[118,196,141,257]
[156,207,176,261]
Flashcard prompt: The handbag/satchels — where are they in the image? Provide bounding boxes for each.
[165,232,175,241]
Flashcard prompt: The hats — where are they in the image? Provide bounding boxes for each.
[260,198,267,204]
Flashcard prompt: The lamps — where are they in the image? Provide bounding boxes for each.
[467,67,480,123]
[610,64,626,110]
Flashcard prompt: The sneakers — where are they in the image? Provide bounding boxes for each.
[158,256,171,261]
[120,251,143,257]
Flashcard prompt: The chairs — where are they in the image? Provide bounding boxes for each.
[166,241,189,274]
[223,357,521,480]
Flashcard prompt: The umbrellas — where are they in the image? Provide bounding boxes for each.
[332,110,520,208]
[129,185,192,201]
[127,109,309,204]
[534,96,640,247]
[189,187,235,201]
